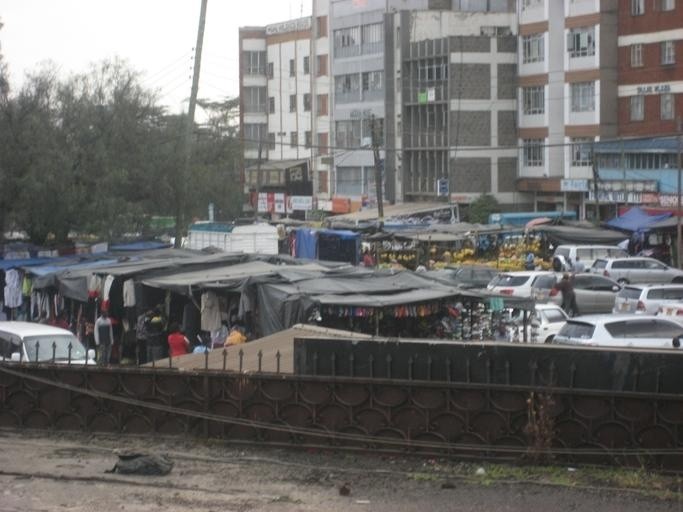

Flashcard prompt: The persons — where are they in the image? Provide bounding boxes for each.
[364,251,372,266]
[495,323,510,342]
[557,273,579,317]
[525,253,535,270]
[168,323,189,356]
[573,256,587,275]
[93,311,115,366]
[416,258,426,272]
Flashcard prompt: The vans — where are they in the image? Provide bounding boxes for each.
[0,320,97,366]
[454,244,683,350]
